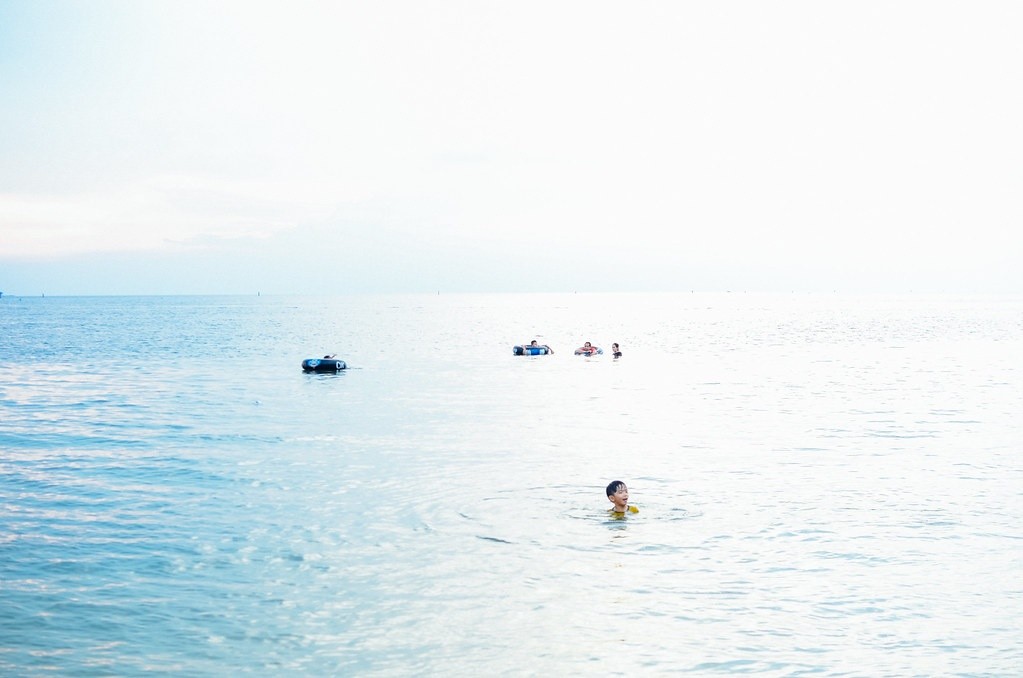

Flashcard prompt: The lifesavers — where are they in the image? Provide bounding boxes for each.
[574,346,603,355]
[512,345,549,355]
[301,359,346,370]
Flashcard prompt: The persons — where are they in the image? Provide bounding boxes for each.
[606,481,638,518]
[522,340,555,354]
[324,353,338,359]
[576,342,598,357]
[612,343,622,359]
